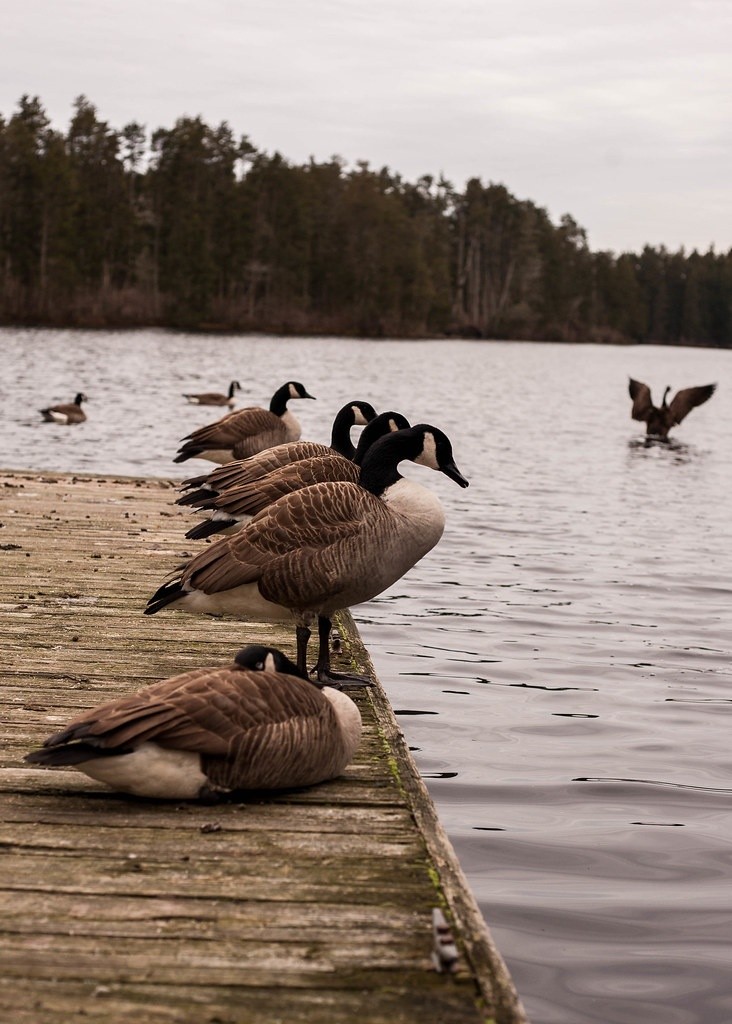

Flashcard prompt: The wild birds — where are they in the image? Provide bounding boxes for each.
[626,374,718,452]
[36,392,90,426]
[23,643,361,799]
[144,381,471,692]
